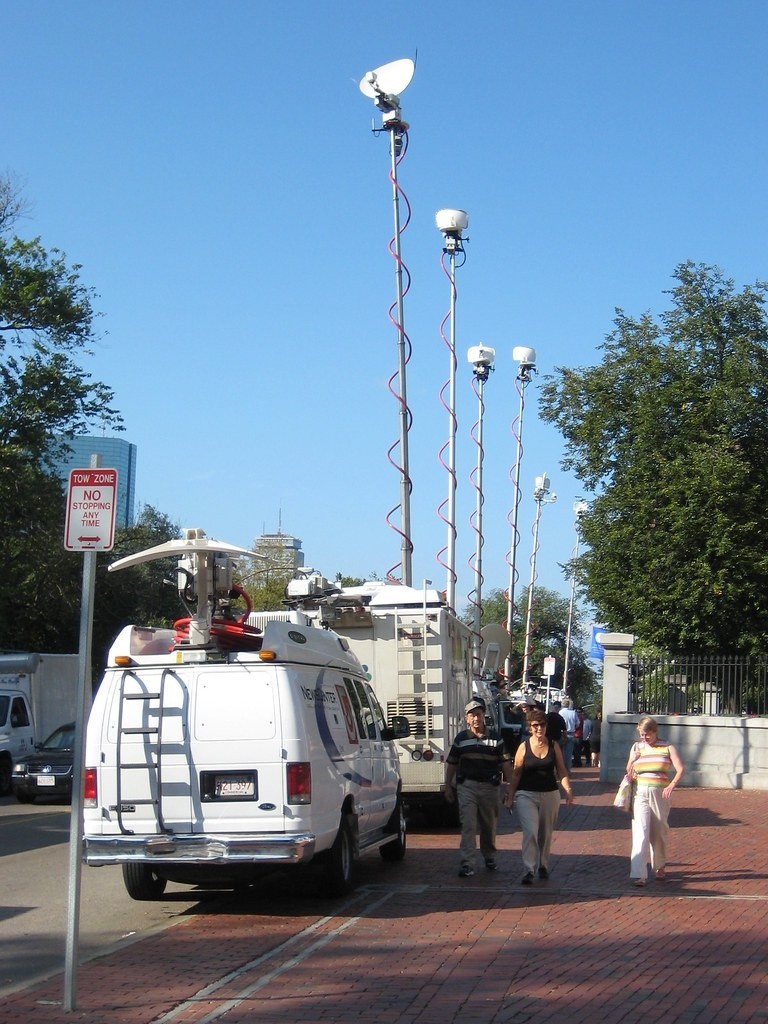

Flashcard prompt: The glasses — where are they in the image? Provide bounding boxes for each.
[530,722,547,728]
[639,732,655,740]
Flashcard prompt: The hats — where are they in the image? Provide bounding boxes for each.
[465,701,486,717]
[553,701,561,708]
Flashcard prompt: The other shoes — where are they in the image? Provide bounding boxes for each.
[463,865,474,876]
[656,865,666,880]
[486,859,498,871]
[538,868,548,879]
[572,759,576,763]
[572,763,583,767]
[634,878,648,886]
[521,873,535,885]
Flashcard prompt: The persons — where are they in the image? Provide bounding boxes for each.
[625,716,686,887]
[444,694,603,886]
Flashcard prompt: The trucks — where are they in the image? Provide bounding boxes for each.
[242,54,574,829]
[1,652,79,799]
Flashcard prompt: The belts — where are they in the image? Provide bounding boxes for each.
[465,777,491,783]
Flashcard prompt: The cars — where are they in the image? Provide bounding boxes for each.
[10,722,75,805]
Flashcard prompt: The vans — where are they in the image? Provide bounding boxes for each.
[82,531,412,903]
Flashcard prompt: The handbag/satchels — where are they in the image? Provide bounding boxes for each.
[613,766,633,813]
